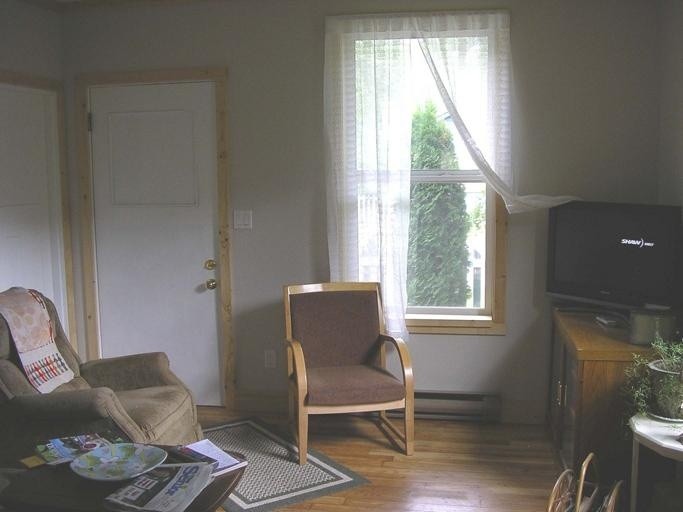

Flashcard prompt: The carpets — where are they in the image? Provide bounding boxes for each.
[203,416,371,512]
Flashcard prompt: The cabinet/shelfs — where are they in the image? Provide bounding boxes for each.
[546,310,683,490]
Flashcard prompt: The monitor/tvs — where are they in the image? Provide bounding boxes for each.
[546,201,683,325]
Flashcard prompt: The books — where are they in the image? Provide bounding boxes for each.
[144,439,249,477]
[34,429,125,472]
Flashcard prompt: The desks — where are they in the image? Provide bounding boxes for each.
[624,413,682,512]
[1,443,247,512]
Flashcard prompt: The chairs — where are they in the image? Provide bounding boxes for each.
[284,281,415,464]
[0,287,199,446]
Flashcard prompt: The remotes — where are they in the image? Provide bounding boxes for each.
[596,315,617,325]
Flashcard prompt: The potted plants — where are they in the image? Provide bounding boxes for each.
[617,332,683,442]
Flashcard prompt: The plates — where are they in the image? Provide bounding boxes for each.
[69,443,168,481]
